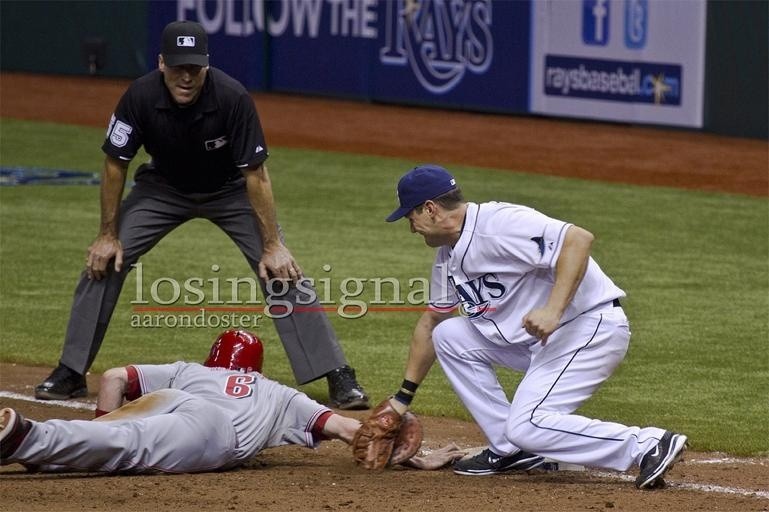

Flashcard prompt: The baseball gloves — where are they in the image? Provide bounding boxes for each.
[352,394,422,472]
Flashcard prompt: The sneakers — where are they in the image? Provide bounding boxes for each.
[0,407,32,459]
[635,429,688,491]
[452,445,559,476]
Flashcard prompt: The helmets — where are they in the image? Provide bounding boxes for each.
[205,328,265,369]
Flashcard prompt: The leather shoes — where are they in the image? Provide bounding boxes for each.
[326,363,372,412]
[35,362,91,402]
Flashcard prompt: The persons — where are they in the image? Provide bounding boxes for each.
[349,161,687,490]
[1,326,466,477]
[30,19,373,411]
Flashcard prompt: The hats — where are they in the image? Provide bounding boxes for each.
[383,165,458,225]
[159,19,209,68]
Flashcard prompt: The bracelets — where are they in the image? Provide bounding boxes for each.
[394,378,419,406]
